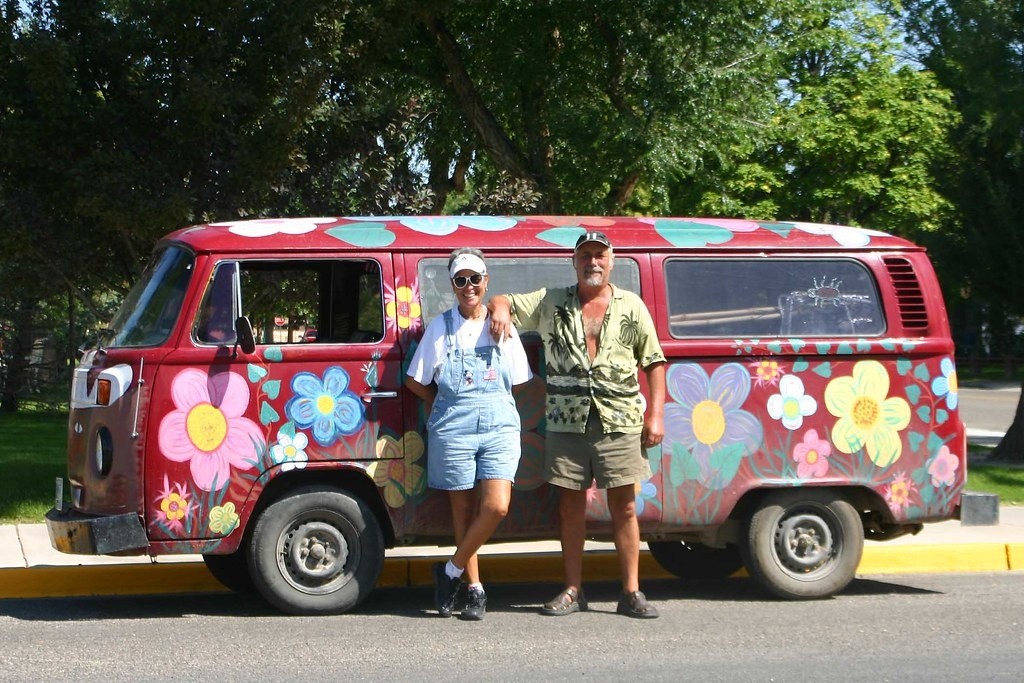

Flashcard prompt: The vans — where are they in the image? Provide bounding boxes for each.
[43,214,966,616]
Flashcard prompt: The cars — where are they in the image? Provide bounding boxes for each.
[297,328,319,343]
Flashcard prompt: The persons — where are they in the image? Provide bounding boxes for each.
[405,247,533,620]
[488,231,667,619]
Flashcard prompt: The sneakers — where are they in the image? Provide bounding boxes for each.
[434,561,461,617]
[460,583,488,620]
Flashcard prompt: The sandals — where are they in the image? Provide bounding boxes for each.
[617,591,659,618]
[539,587,588,615]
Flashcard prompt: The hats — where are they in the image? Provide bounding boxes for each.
[450,254,486,280]
[575,232,611,252]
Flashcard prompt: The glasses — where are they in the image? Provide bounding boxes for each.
[452,273,485,289]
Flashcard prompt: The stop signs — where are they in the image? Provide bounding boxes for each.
[275,316,285,326]
